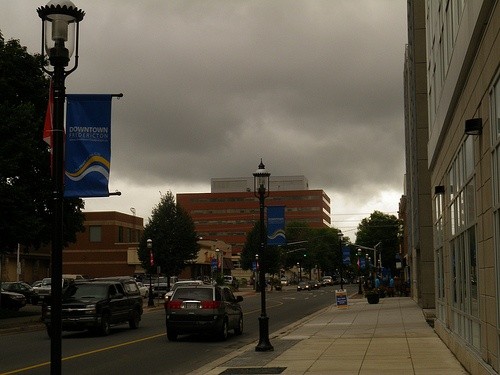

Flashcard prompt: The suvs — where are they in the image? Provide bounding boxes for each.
[223,276,233,285]
[164,279,205,308]
[39,274,143,338]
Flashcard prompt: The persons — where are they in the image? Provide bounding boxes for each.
[375,274,399,294]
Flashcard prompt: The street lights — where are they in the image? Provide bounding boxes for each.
[296,262,300,284]
[252,158,274,352]
[146,238,154,306]
[36,0,86,375]
[215,247,220,284]
[255,254,259,292]
[337,233,345,291]
[357,248,362,294]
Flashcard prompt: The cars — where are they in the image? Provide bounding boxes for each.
[296,276,350,292]
[128,281,174,299]
[165,284,244,342]
[0,274,85,314]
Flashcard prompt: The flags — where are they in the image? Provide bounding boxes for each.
[43,79,65,179]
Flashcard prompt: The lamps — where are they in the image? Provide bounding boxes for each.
[465,117,483,135]
[435,185,444,195]
